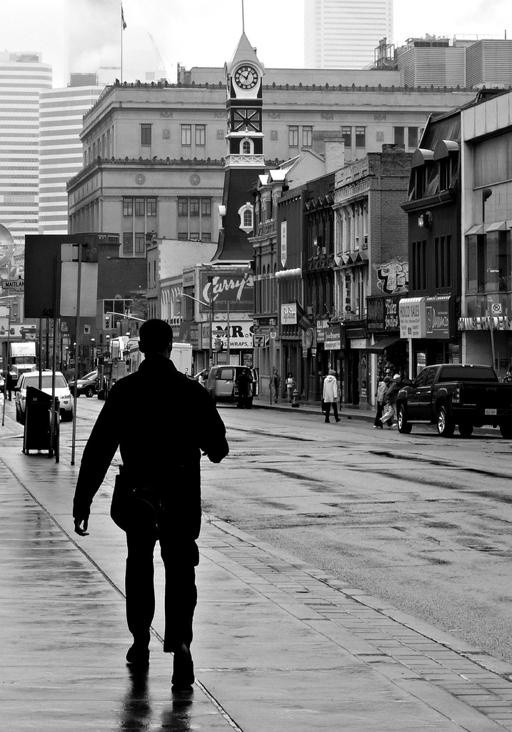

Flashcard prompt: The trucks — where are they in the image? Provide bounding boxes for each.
[4,341,36,390]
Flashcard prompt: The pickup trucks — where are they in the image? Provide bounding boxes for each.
[396,364,512,438]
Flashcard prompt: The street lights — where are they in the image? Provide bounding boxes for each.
[105,311,146,323]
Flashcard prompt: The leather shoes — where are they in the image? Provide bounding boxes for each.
[170,644,197,690]
[125,643,150,663]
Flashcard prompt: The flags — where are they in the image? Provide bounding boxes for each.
[121,6,127,30]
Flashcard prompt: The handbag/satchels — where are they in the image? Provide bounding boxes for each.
[111,466,163,538]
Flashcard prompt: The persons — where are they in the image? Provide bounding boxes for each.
[269,368,280,404]
[372,376,396,428]
[322,370,340,424]
[285,372,295,403]
[377,373,403,428]
[72,318,230,687]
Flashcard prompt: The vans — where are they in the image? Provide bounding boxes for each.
[211,364,259,408]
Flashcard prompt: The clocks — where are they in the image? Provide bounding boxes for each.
[234,65,258,89]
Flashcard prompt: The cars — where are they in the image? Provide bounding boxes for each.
[68,371,104,397]
[13,372,74,422]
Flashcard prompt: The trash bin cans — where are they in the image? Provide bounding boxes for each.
[20,386,59,456]
